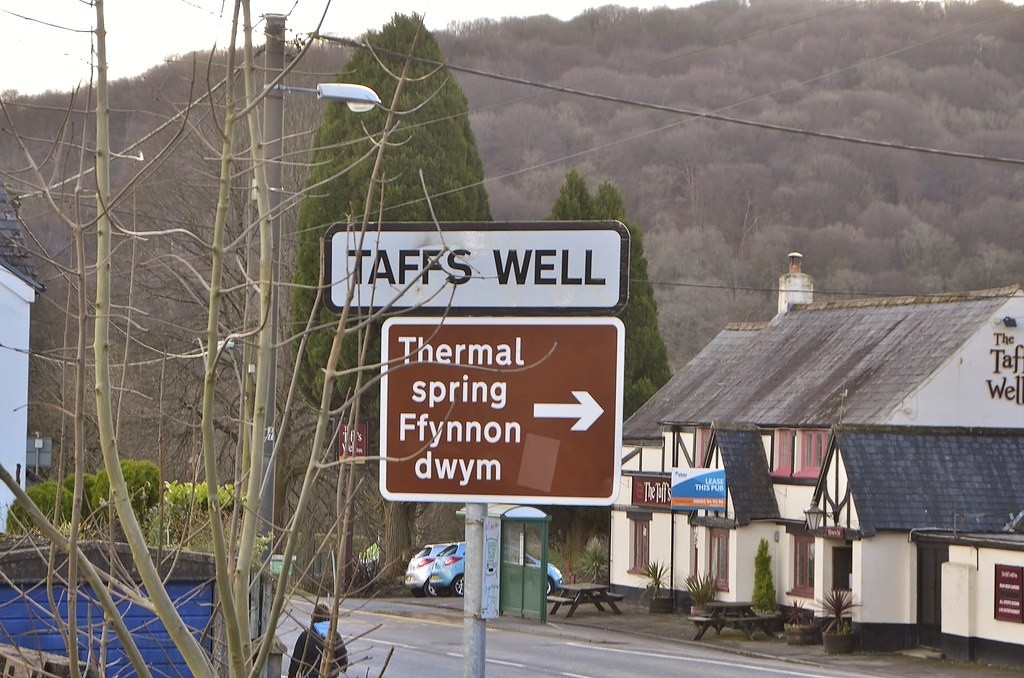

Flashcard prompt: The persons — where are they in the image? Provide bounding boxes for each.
[288,603,348,678]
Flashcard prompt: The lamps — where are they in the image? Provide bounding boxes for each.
[804,495,840,531]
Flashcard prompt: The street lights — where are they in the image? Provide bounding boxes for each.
[251,82,382,638]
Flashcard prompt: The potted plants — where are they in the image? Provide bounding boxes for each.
[640,560,676,613]
[784,600,820,644]
[584,536,609,584]
[687,574,720,616]
[817,588,863,655]
[750,540,781,631]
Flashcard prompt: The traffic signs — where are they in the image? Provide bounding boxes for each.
[379,316,625,506]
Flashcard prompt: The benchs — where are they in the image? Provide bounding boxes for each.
[688,613,781,629]
[545,592,629,605]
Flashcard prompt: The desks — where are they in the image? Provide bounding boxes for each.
[691,600,774,644]
[548,583,622,617]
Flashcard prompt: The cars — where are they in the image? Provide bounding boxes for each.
[430,541,564,597]
[405,543,457,597]
